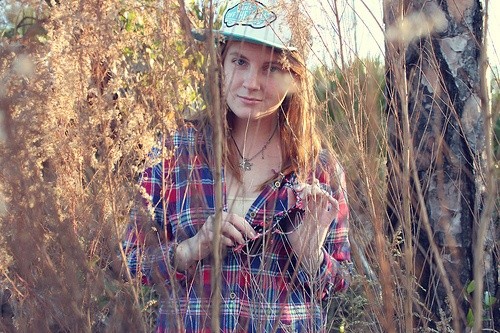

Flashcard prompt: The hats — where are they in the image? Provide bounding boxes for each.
[190,0,313,63]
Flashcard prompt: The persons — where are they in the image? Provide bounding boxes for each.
[125,1,353,333]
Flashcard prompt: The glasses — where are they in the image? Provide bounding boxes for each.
[233,169,307,258]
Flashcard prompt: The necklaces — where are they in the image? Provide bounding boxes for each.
[228,115,279,172]
